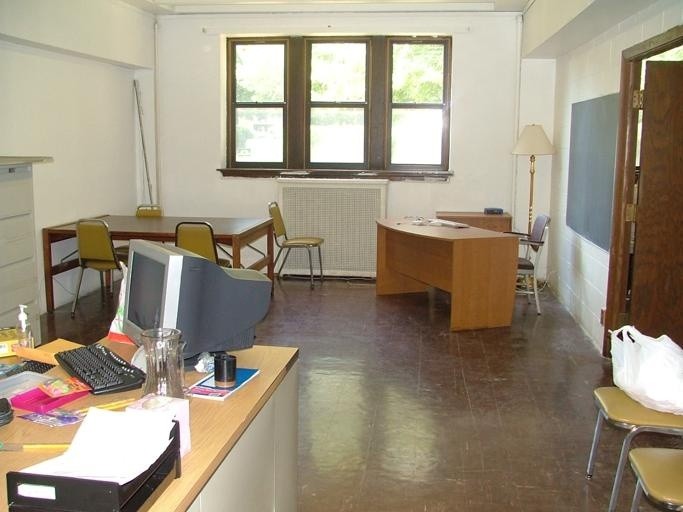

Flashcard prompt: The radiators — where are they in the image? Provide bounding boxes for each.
[275,178,389,282]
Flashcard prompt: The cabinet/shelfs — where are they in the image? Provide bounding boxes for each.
[0,163,42,349]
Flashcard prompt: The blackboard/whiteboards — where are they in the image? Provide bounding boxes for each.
[566,90,620,254]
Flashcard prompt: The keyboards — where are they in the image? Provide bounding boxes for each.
[54,345,145,394]
[429,218,469,228]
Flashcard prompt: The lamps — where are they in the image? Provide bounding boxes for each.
[513,125,556,290]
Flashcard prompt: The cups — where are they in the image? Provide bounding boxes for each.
[214,355,236,389]
[177,340,185,388]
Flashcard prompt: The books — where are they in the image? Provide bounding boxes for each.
[181,367,259,400]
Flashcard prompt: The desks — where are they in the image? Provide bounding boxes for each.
[42,215,275,314]
[375,218,519,333]
[435,211,513,233]
[0,336,300,512]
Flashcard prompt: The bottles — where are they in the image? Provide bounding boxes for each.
[139,327,186,400]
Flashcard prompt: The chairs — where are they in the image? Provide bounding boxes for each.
[585,387,683,512]
[268,201,324,290]
[175,222,231,270]
[627,447,683,512]
[503,213,552,316]
[134,202,165,215]
[71,219,128,318]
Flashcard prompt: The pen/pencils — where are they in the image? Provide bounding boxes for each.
[23,444,70,448]
[75,398,137,417]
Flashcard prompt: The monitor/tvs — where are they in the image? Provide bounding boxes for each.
[123,239,272,372]
